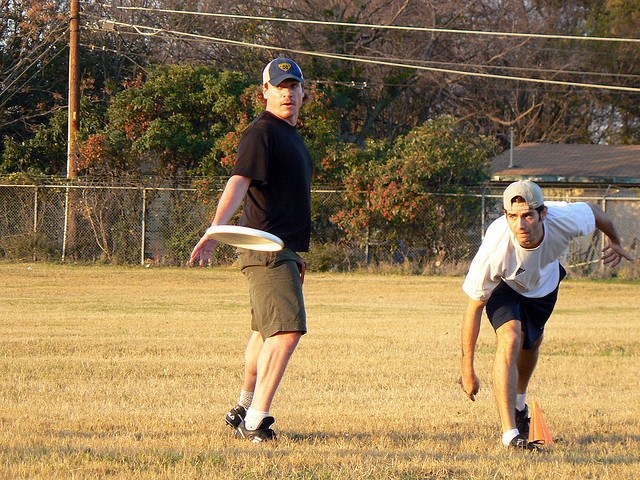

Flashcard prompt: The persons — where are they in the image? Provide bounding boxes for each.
[459,180,632,444]
[190,58,313,444]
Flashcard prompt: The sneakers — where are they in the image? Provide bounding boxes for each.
[225,404,246,429]
[515,403,529,439]
[508,434,546,453]
[238,415,276,444]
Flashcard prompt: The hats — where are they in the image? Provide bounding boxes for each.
[262,57,303,87]
[503,180,544,210]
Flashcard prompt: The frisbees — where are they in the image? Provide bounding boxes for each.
[206,225,284,252]
